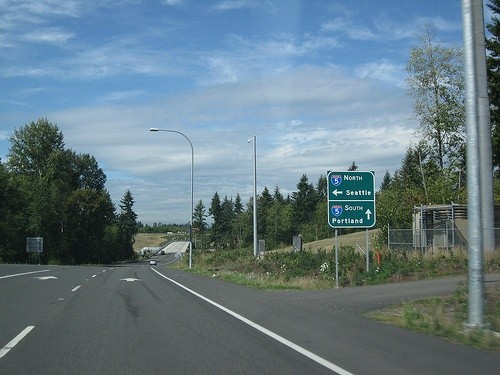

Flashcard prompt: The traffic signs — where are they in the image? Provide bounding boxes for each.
[327,170,376,229]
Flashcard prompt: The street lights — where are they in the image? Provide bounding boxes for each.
[246,134,258,257]
[149,126,193,271]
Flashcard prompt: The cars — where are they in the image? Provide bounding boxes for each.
[150,260,157,265]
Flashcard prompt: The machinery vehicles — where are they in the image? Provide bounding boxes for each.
[161,251,165,255]
